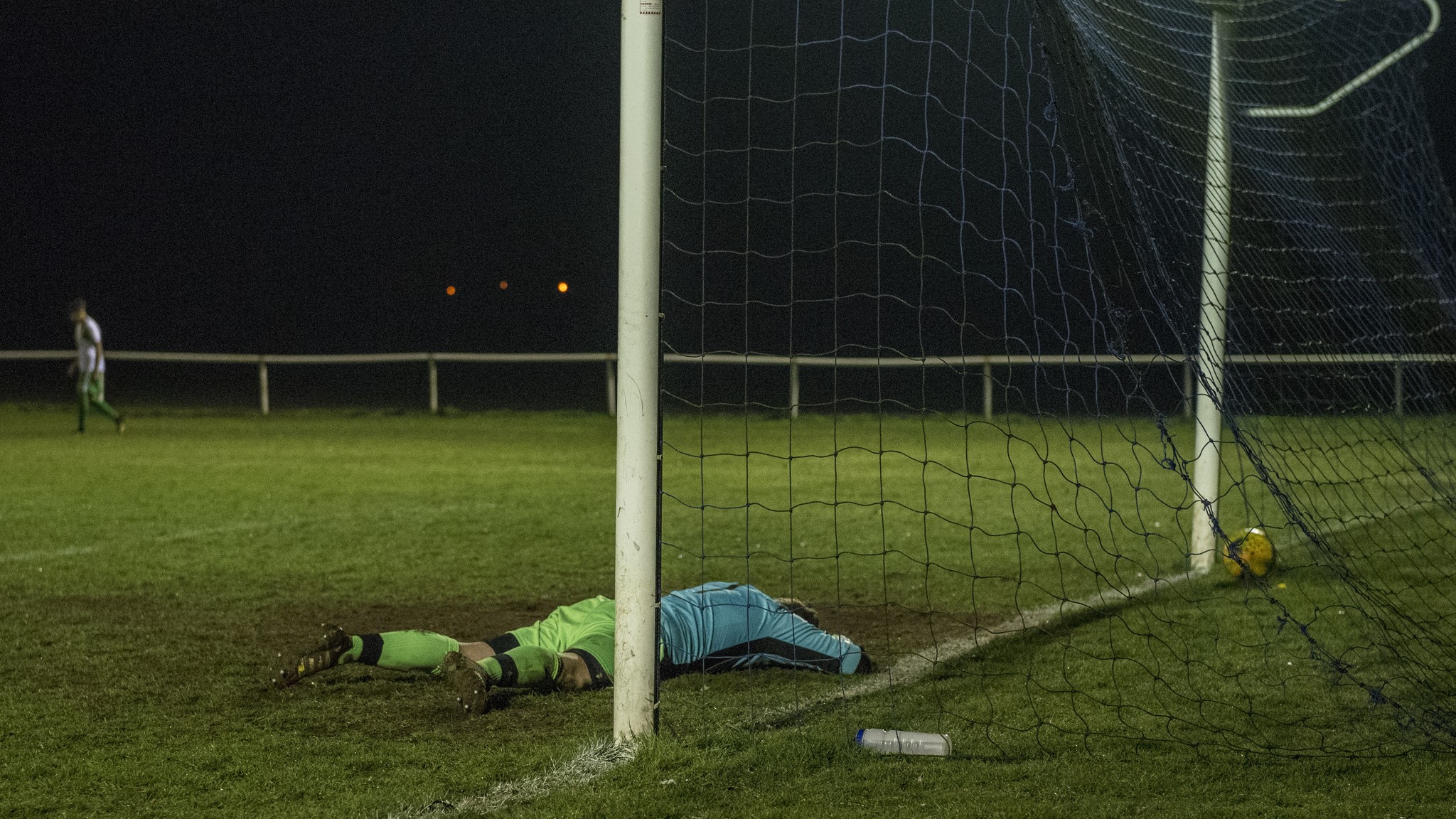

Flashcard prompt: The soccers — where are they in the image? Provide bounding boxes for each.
[1223,528,1274,579]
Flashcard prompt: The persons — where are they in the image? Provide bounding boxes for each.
[266,579,873,718]
[65,297,131,439]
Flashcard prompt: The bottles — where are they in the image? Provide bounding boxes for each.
[856,729,952,757]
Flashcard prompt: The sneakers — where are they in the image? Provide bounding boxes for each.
[269,622,352,686]
[442,650,489,713]
[117,414,128,435]
